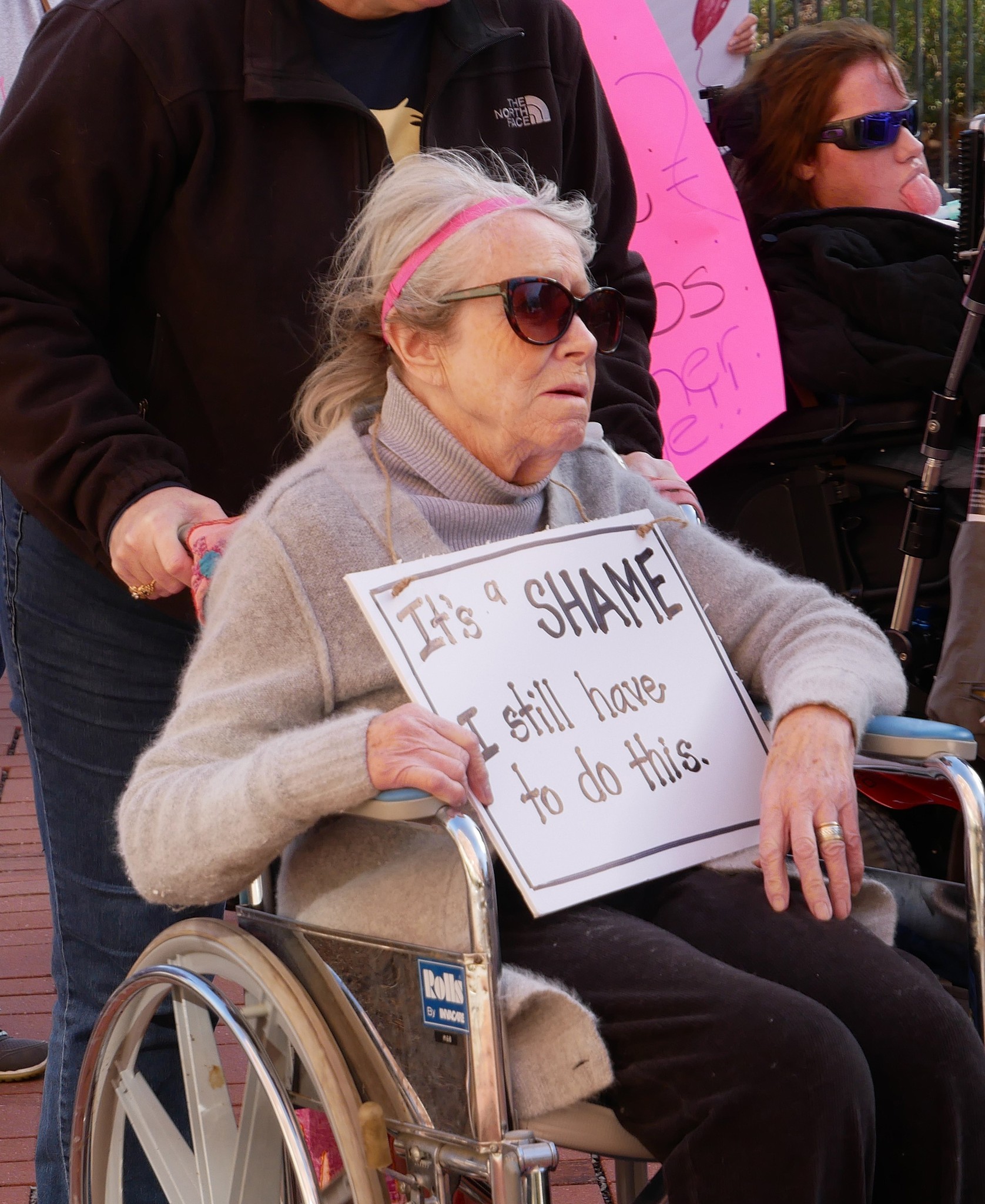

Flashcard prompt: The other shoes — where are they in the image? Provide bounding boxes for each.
[1,1029,50,1083]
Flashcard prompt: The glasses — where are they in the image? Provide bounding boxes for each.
[437,276,627,354]
[814,99,922,151]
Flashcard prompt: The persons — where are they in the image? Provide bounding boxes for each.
[0,0,687,1204]
[711,16,985,361]
[0,1027,48,1082]
[110,147,985,1204]
[726,13,760,57]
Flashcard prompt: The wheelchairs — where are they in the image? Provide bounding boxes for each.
[67,517,985,1204]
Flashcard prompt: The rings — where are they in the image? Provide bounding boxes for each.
[129,579,155,600]
[754,41,761,50]
[818,822,848,844]
